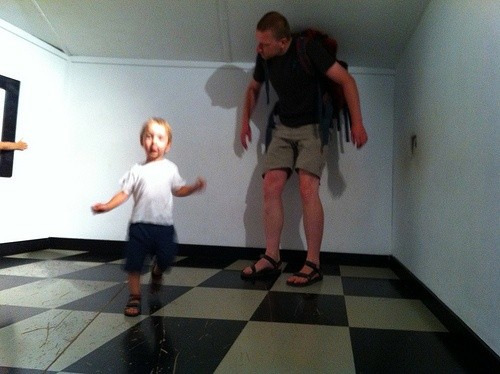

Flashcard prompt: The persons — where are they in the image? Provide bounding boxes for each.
[240,10,368,287]
[91,117,206,317]
[0,139,28,151]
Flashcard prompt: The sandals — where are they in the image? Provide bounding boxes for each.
[123,292,143,317]
[285,260,326,287]
[241,252,283,279]
[150,268,166,293]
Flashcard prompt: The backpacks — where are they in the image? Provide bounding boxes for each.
[264,30,352,154]
